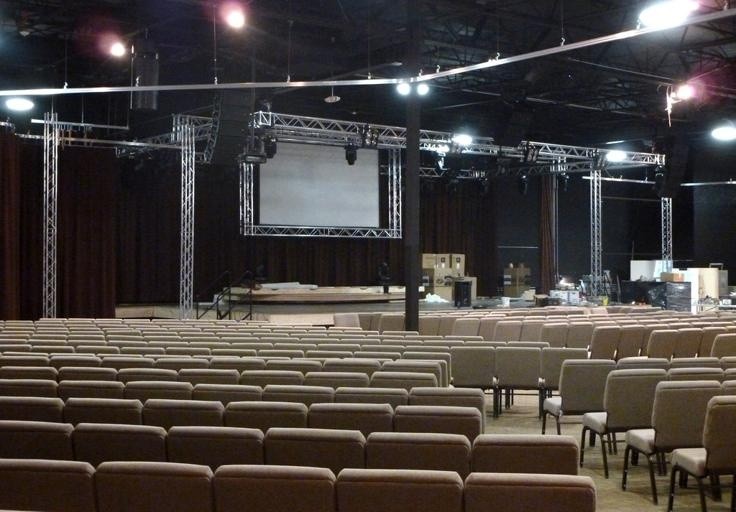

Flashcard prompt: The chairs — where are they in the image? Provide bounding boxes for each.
[0,298,736,512]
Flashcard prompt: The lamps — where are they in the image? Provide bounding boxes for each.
[265,133,279,160]
[342,141,360,164]
[431,135,672,198]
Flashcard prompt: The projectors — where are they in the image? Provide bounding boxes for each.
[244,153,266,164]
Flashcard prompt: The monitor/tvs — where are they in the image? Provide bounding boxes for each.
[708,262,723,270]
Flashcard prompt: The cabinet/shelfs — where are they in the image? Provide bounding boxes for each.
[502,267,531,296]
[420,267,452,301]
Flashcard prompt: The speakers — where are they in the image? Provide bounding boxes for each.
[454,281,471,306]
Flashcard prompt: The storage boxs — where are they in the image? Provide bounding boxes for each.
[660,271,684,283]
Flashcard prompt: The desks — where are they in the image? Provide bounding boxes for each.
[620,278,692,312]
